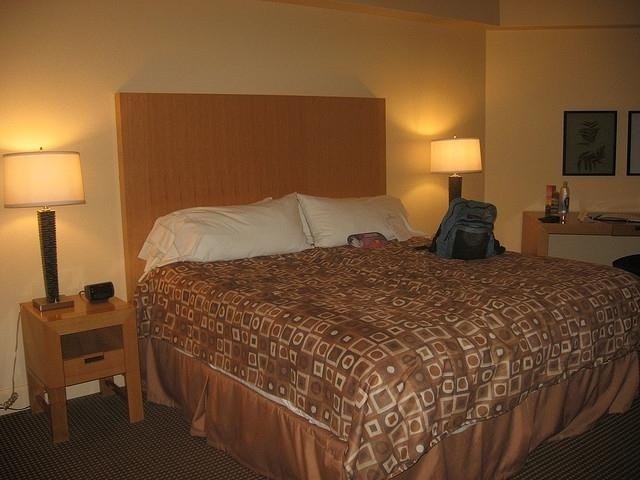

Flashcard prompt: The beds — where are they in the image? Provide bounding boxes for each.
[137,236,638,479]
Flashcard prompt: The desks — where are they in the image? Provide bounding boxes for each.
[522,209,639,266]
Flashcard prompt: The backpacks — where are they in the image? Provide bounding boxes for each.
[429,197,497,260]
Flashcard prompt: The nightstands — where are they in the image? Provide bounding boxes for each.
[20,291,145,446]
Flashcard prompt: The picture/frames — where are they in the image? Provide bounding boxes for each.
[563,109,640,176]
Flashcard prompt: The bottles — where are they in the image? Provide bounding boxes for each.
[558,181,570,216]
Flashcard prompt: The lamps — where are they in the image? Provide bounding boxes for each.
[429,133,483,214]
[3,145,89,312]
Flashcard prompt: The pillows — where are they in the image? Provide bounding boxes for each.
[137,188,416,266]
